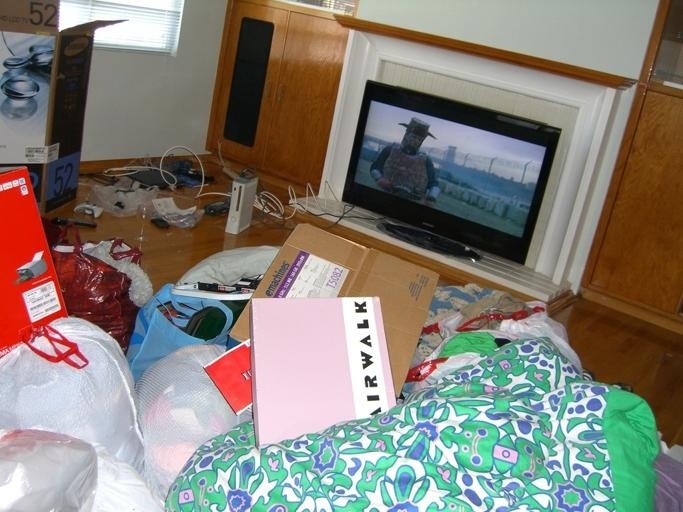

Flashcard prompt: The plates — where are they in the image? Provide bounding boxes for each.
[0,76,40,102]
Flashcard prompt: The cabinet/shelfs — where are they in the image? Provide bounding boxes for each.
[207,1,347,195]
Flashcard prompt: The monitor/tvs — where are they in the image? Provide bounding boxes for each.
[341,79,563,266]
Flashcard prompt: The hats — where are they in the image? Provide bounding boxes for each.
[396,118,438,142]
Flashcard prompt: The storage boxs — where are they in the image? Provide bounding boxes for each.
[0,0,131,218]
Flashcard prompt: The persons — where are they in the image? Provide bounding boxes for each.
[370,117,440,206]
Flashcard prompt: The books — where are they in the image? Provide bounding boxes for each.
[249,297,397,447]
